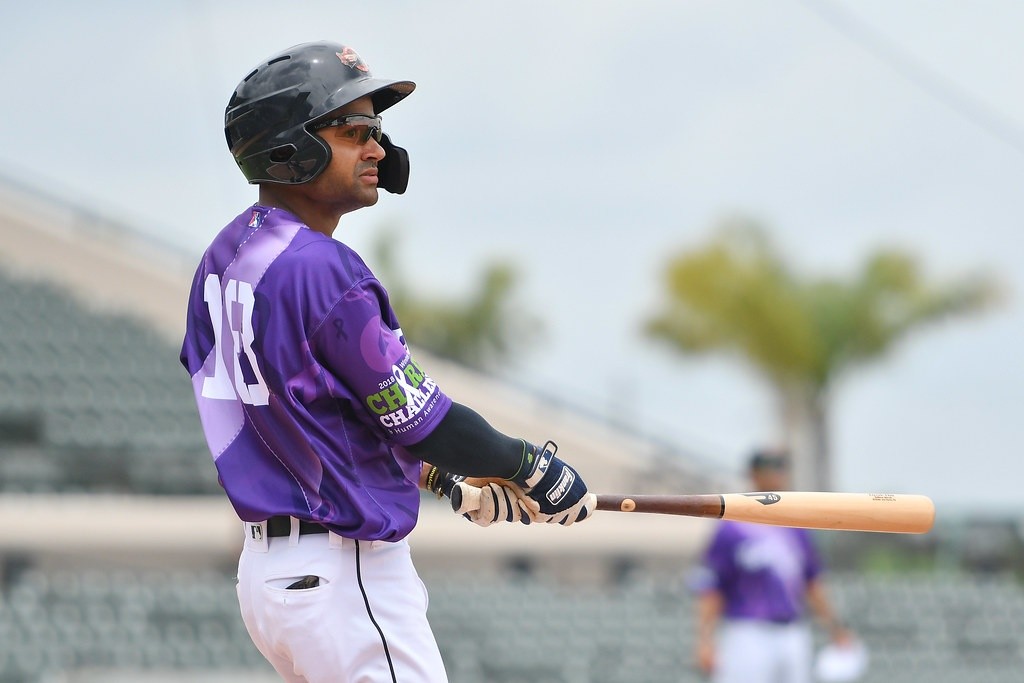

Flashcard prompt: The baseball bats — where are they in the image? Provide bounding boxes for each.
[450,480,937,536]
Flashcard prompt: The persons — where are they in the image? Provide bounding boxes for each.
[180,40,593,683]
[695,450,855,683]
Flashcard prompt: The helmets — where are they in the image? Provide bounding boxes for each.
[223,40,417,195]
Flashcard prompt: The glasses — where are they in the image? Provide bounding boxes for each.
[310,113,383,146]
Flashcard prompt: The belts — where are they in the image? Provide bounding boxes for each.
[267,515,329,537]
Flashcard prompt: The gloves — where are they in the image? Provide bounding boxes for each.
[425,437,595,527]
